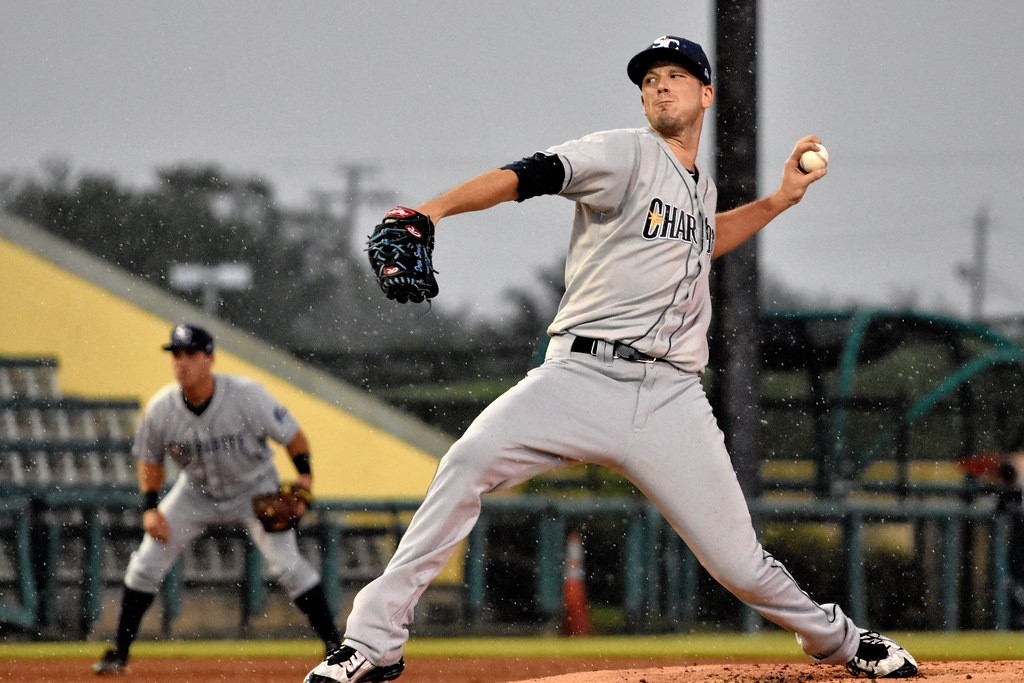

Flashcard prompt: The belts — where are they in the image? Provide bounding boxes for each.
[571,336,668,363]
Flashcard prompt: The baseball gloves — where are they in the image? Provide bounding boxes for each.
[252,483,314,533]
[367,205,439,304]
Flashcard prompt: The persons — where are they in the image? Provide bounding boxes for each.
[92,324,345,676]
[301,35,920,683]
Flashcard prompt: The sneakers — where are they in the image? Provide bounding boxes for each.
[839,627,918,678]
[92,649,127,674]
[324,645,341,660]
[302,645,405,683]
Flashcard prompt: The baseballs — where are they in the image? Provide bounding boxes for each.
[799,141,829,173]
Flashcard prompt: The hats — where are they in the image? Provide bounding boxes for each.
[162,324,213,352]
[627,35,712,86]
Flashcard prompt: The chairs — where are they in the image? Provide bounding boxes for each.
[0,356,399,595]
